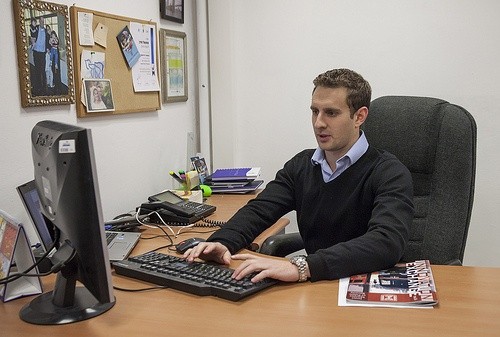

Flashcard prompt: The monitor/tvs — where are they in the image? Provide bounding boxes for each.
[19,120,117,325]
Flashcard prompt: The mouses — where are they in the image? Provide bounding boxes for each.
[176,238,206,253]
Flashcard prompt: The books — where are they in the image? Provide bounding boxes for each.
[346,260,439,307]
[203,167,265,195]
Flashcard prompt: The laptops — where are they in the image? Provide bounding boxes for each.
[17,180,142,263]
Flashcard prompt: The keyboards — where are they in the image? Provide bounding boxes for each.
[111,252,277,302]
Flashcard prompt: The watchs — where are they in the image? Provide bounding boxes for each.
[290,254,308,282]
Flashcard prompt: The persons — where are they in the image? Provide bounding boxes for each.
[183,68,413,283]
[29,16,61,95]
[89,86,108,109]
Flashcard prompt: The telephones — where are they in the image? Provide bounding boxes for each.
[136,191,218,224]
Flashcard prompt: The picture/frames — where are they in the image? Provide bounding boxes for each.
[159,29,189,104]
[159,0,184,23]
[13,0,75,108]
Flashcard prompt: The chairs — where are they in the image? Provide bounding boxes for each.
[260,96,477,265]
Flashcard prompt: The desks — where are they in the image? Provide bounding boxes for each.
[0,189,500,337]
